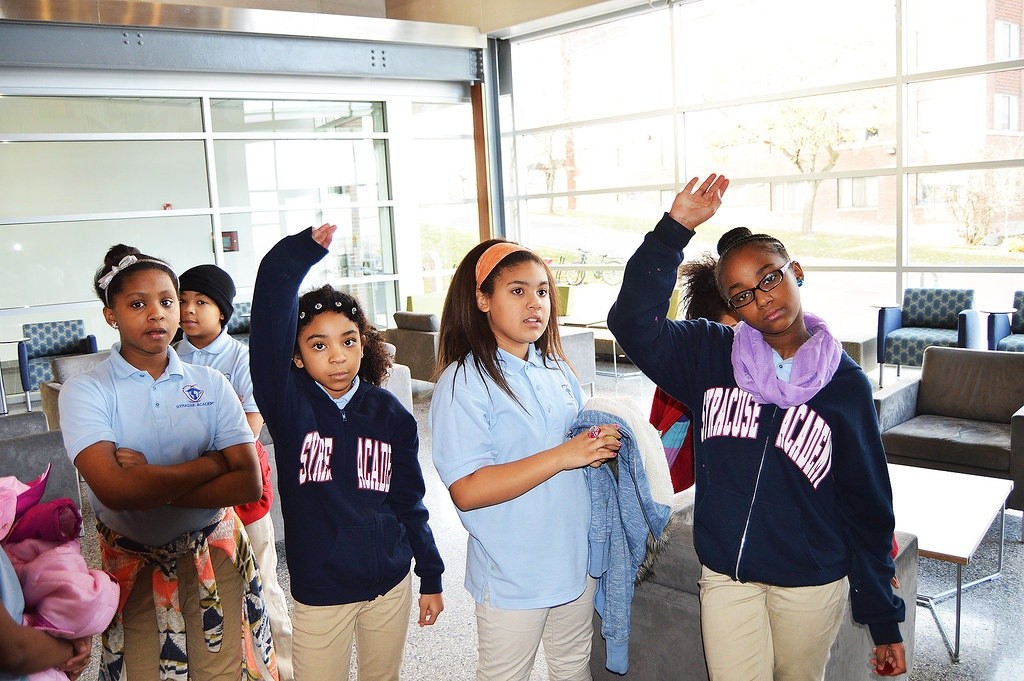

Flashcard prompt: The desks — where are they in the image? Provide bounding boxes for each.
[0,338,30,414]
[558,325,595,397]
[888,464,1015,662]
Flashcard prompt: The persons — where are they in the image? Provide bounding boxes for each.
[57,244,279,681]
[428,239,623,681]
[0,546,93,681]
[647,258,742,498]
[248,223,446,681]
[171,264,294,681]
[608,174,906,681]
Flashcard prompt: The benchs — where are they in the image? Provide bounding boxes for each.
[555,286,681,379]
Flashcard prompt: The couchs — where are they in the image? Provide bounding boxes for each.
[590,490,917,681]
[378,312,441,417]
[876,288,979,388]
[872,345,1024,544]
[225,302,273,445]
[0,320,111,537]
[987,291,1024,351]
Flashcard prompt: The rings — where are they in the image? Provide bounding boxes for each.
[588,426,601,439]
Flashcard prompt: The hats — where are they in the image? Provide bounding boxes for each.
[179,264,236,328]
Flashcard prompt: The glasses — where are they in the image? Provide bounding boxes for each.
[726,260,791,310]
